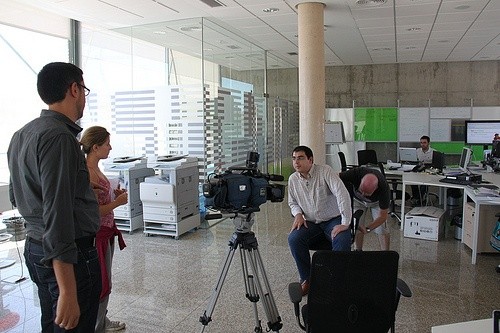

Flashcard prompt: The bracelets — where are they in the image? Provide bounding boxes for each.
[113,200,120,206]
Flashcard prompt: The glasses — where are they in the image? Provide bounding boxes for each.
[78,83,90,96]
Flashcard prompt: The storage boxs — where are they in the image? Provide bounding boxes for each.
[404,206,447,242]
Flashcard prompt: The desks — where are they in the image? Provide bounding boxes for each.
[372,163,500,264]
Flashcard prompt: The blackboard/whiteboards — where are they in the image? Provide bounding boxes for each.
[325,121,343,144]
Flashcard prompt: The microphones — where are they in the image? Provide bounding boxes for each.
[264,174,285,181]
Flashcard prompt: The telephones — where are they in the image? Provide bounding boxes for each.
[412,163,426,172]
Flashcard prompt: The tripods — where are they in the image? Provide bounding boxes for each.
[198,212,282,333]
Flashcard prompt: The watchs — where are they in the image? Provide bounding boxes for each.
[364,226,371,233]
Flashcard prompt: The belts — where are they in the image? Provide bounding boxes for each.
[25,234,96,250]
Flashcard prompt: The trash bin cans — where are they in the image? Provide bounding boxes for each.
[447,188,460,205]
[454,215,462,239]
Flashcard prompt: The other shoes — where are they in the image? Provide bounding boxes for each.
[300,280,311,296]
[410,197,424,205]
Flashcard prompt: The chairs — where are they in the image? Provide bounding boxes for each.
[337,150,443,228]
[288,251,410,333]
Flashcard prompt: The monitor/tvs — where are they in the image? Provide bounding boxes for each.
[459,147,471,172]
[399,147,417,164]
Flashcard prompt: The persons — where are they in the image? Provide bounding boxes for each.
[79,126,128,333]
[340,166,391,251]
[7,62,101,333]
[411,136,437,207]
[287,146,352,296]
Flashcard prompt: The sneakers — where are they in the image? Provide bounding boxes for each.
[104,316,125,330]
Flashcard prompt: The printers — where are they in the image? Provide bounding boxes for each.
[136,153,200,223]
[102,156,155,217]
